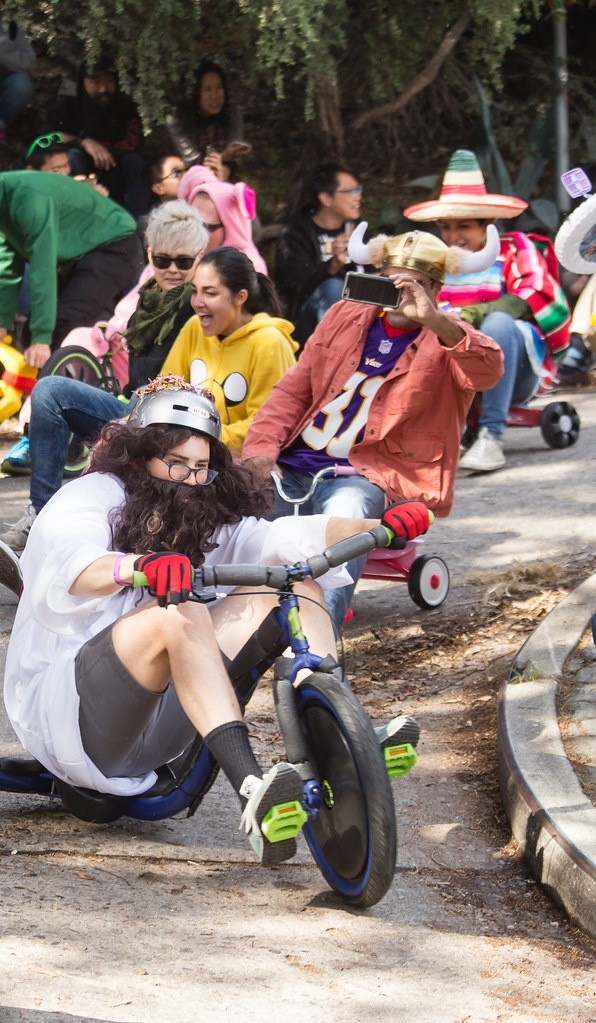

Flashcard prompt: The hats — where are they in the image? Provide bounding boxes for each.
[402,150,529,223]
[348,222,500,284]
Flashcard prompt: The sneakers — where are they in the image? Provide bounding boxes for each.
[0,540,24,594]
[1,436,31,475]
[0,504,39,550]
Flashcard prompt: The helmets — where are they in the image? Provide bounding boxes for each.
[126,388,221,441]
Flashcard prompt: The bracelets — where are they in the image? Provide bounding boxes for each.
[113,553,133,586]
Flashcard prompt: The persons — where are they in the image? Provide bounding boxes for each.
[239,229,505,639]
[0,24,596,868]
[272,162,370,357]
[164,60,253,182]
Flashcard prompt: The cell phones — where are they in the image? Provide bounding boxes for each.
[341,272,405,307]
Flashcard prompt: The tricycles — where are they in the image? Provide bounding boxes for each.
[33,319,130,479]
[468,391,581,448]
[0,464,451,908]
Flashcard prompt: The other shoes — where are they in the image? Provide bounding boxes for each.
[457,426,504,470]
[373,714,420,751]
[557,341,591,376]
[238,763,304,863]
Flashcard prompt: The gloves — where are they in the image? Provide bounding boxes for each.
[381,500,429,550]
[134,551,191,607]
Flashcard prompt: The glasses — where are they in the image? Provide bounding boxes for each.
[151,250,203,271]
[203,223,223,231]
[160,168,189,181]
[153,454,218,485]
[335,185,362,197]
[25,132,63,160]
